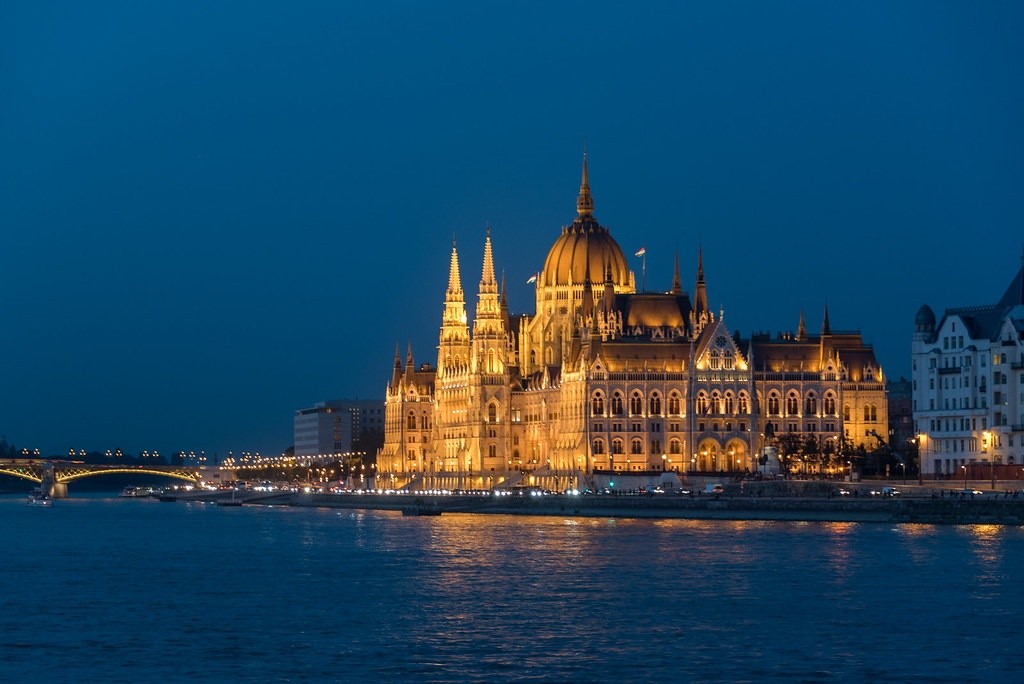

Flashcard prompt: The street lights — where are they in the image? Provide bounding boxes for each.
[988,345,1004,489]
[917,433,925,485]
[758,433,767,478]
[846,460,853,481]
[900,463,907,484]
[832,434,844,466]
[311,448,748,482]
[961,464,968,488]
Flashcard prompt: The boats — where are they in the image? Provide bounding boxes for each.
[117,483,178,502]
[26,487,54,506]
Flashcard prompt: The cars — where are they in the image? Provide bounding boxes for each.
[832,488,901,498]
[203,477,301,492]
[973,489,983,495]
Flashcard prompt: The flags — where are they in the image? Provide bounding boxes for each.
[635,247,645,257]
[527,275,536,284]
